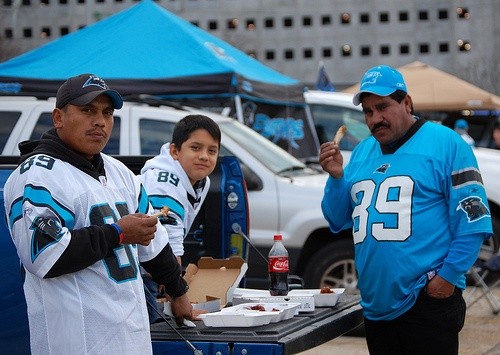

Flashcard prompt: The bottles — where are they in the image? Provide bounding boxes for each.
[267,234,289,296]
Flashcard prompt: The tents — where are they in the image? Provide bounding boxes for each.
[0,0,320,154]
[339,61,500,123]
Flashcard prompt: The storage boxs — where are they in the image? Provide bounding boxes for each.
[163,256,248,318]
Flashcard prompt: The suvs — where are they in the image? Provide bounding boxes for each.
[0,91,366,337]
[173,90,500,288]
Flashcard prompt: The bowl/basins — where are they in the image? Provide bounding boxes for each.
[195,286,346,327]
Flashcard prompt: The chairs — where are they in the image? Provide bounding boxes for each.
[464,247,500,315]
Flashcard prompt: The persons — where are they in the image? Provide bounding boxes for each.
[136,114,221,322]
[4,74,193,355]
[455,119,475,147]
[320,65,493,355]
[493,126,500,150]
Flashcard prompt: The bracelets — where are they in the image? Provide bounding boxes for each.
[112,222,124,245]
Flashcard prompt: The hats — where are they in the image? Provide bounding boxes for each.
[56,74,123,109]
[353,66,407,106]
[456,120,469,130]
